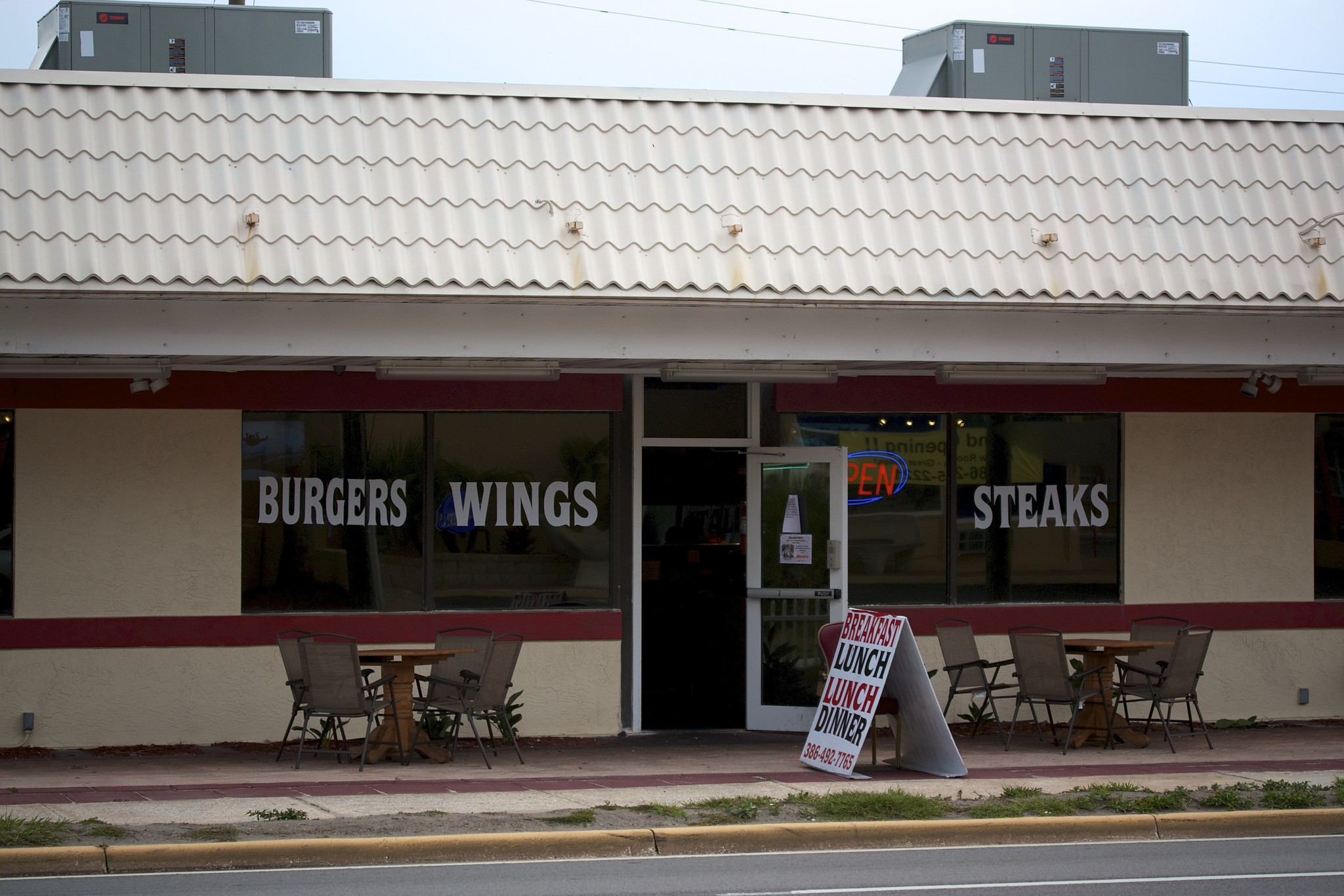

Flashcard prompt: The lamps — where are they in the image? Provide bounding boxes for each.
[0,356,172,380]
[659,361,839,384]
[129,378,150,393]
[1260,371,1282,394]
[936,366,1108,386]
[149,378,170,393]
[373,360,562,382]
[1239,371,1258,397]
[1295,367,1344,386]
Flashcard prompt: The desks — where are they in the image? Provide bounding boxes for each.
[350,647,480,764]
[1053,639,1177,749]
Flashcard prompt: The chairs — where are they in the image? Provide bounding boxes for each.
[410,632,525,769]
[934,619,1044,751]
[274,627,383,765]
[1002,625,1115,755]
[817,620,903,772]
[418,626,499,762]
[293,633,406,773]
[1116,615,1194,742]
[1104,625,1213,754]
